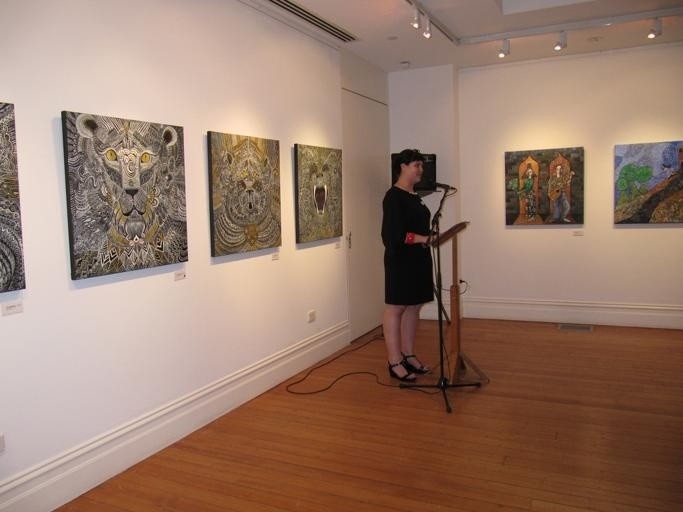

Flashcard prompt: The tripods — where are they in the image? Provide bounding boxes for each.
[399,189,481,413]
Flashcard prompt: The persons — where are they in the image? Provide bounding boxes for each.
[377,148,443,384]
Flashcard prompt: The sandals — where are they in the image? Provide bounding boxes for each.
[401,352,430,376]
[387,359,416,382]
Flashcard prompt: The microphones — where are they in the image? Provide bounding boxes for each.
[426,180,455,189]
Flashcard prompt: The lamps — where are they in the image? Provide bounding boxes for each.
[499,9,661,58]
[411,6,432,39]
